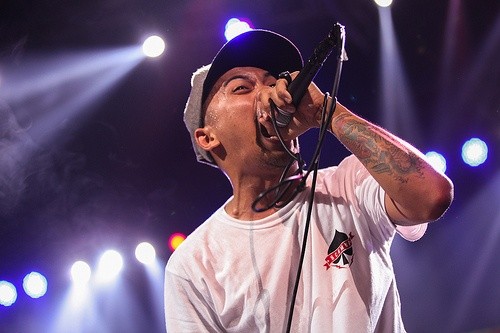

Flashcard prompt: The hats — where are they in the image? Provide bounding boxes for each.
[182,29,305,168]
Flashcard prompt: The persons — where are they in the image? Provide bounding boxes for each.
[161,28,456,333]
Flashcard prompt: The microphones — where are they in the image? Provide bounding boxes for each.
[269,23,343,128]
